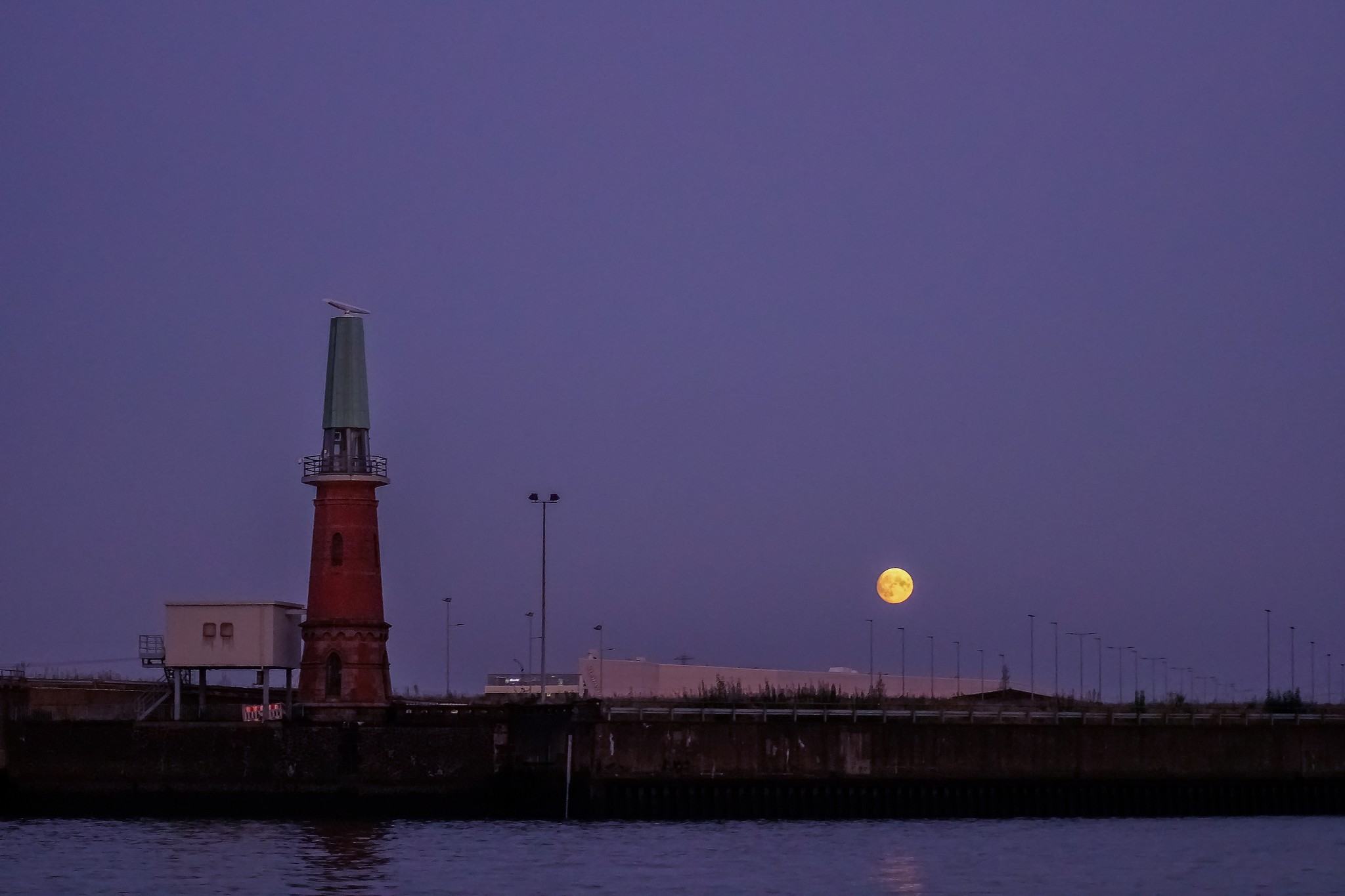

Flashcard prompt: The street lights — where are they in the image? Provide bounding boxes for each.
[1194,676,1253,705]
[1093,637,1102,704]
[1050,622,1058,705]
[528,492,560,700]
[442,597,465,703]
[927,636,934,699]
[1107,646,1135,705]
[1131,650,1138,705]
[1341,664,1345,703]
[525,611,542,699]
[999,654,1007,691]
[1170,666,1194,696]
[1264,608,1272,700]
[1309,641,1317,703]
[1066,632,1096,702]
[977,649,984,702]
[865,619,874,696]
[1326,653,1332,704]
[594,625,616,698]
[1140,656,1166,704]
[897,627,905,698]
[1027,614,1036,706]
[1289,626,1296,694]
[953,641,960,696]
